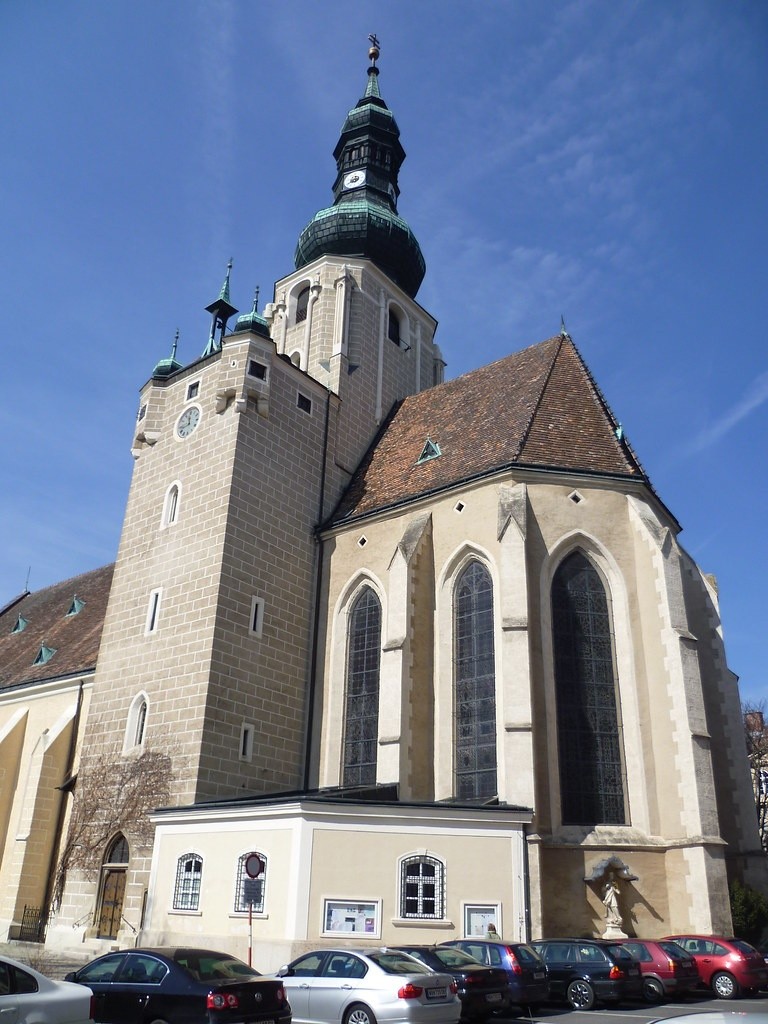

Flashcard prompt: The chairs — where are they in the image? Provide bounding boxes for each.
[464,947,472,955]
[331,959,345,977]
[479,947,491,963]
[130,963,147,978]
[689,942,697,952]
[455,957,464,965]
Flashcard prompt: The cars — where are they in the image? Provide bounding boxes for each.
[332,946,524,1024]
[0,955,99,1024]
[526,938,644,1011]
[392,944,513,1022]
[418,939,552,1014]
[661,934,768,999]
[60,947,293,1024]
[608,939,700,1003]
[263,946,461,1024]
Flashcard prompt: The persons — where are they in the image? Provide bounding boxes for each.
[485,923,501,940]
[603,880,623,927]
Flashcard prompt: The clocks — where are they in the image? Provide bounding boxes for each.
[344,171,364,188]
[177,406,199,438]
